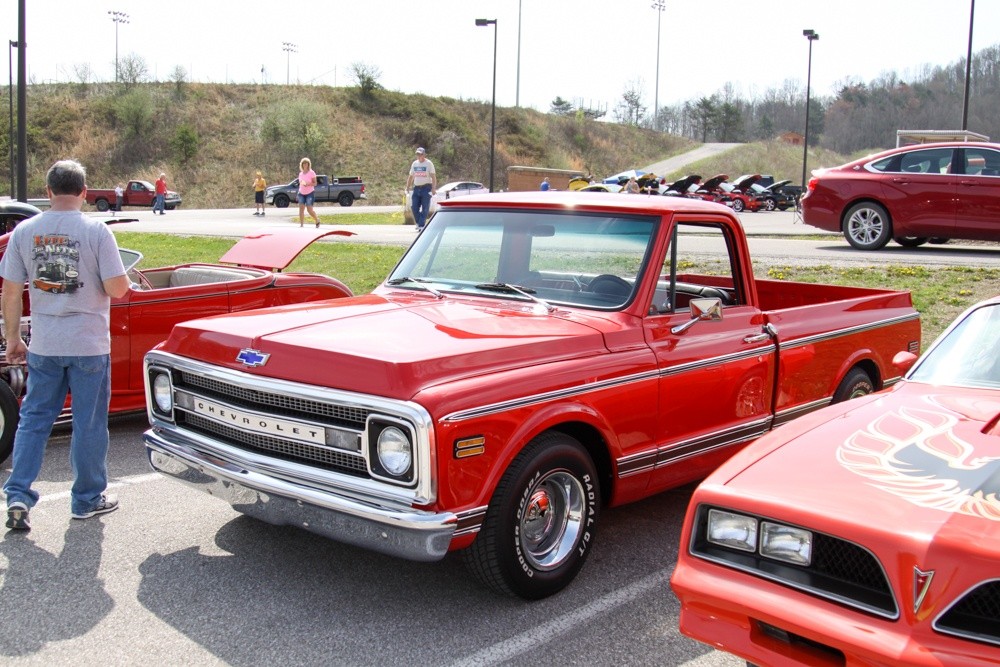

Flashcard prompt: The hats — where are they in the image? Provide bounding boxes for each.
[415,147,425,154]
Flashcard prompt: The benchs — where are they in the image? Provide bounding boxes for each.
[168,267,252,292]
[507,268,729,308]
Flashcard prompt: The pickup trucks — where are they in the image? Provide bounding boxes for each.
[264,173,367,207]
[143,188,921,601]
[83,180,183,211]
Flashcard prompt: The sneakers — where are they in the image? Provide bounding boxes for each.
[5,501,32,529]
[72,495,118,518]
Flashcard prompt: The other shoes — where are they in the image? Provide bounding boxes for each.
[259,212,266,217]
[252,212,260,216]
[418,227,424,232]
[152,209,156,214]
[299,226,304,228]
[415,223,419,230]
[160,213,166,215]
[316,218,321,228]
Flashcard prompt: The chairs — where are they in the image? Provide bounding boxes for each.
[588,272,632,306]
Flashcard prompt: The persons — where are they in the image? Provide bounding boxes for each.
[0,159,131,529]
[643,172,659,195]
[589,175,596,186]
[540,177,550,191]
[152,173,166,215]
[6,218,16,232]
[627,177,640,194]
[252,172,267,216]
[115,183,123,211]
[405,147,437,232]
[296,158,320,228]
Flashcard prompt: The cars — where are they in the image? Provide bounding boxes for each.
[672,294,999,667]
[433,181,489,203]
[800,141,1000,251]
[567,169,808,214]
[0,194,45,258]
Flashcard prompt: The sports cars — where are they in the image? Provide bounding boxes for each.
[0,214,360,465]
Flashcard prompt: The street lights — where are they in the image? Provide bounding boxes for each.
[800,29,820,198]
[281,42,297,84]
[649,0,667,133]
[475,17,497,191]
[107,10,131,82]
